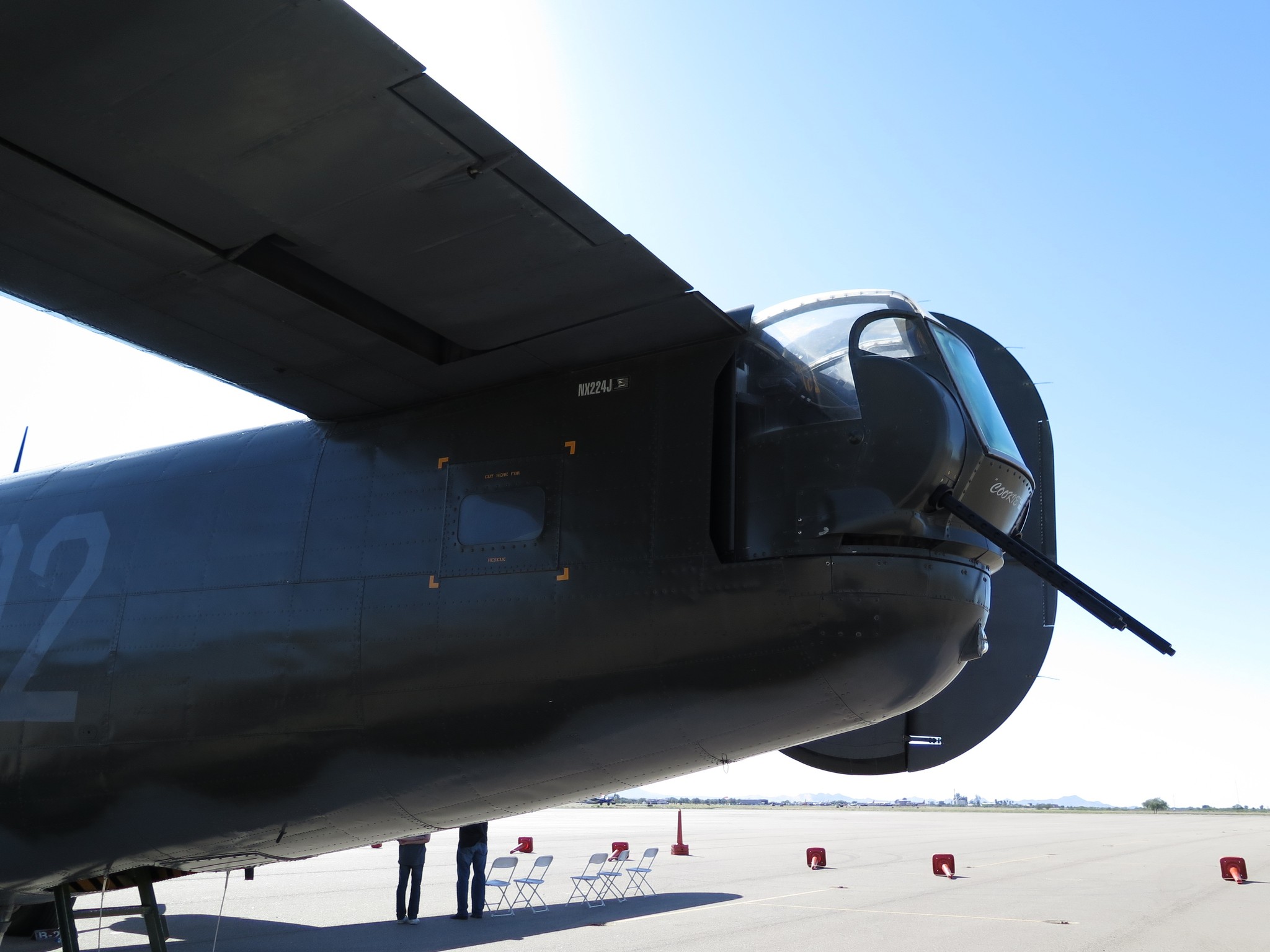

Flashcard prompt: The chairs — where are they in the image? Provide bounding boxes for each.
[508,855,553,914]
[564,853,608,909]
[622,847,659,899]
[483,857,518,918]
[594,850,630,904]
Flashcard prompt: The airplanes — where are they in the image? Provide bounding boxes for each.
[717,796,1033,807]
[574,793,620,806]
[0,0,1177,952]
[642,797,670,804]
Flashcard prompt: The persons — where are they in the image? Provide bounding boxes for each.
[451,820,488,920]
[395,833,431,925]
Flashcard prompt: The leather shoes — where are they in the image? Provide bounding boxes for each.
[450,915,468,920]
[469,914,482,918]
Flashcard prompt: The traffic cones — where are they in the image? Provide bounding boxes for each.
[510,836,533,854]
[1220,855,1248,884]
[932,854,956,880]
[607,841,629,861]
[806,847,827,870]
[671,807,689,856]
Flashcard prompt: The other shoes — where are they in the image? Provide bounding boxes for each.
[396,915,407,923]
[408,917,420,924]
